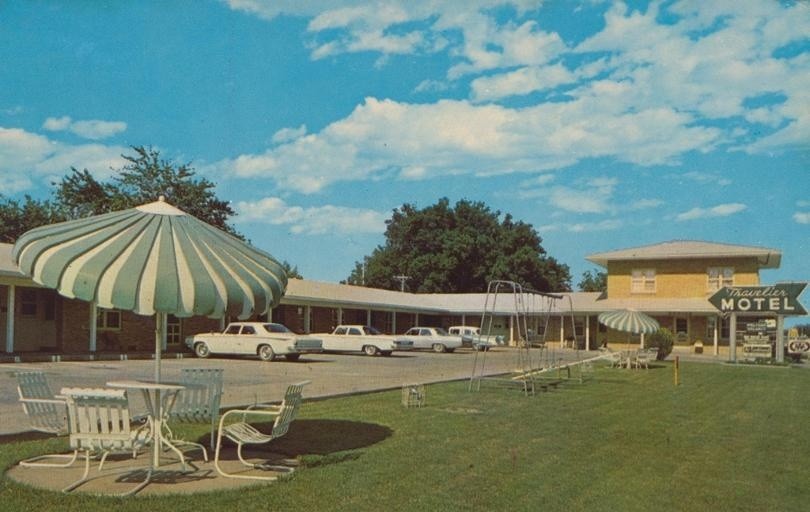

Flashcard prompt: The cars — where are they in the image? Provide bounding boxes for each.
[309,324,506,356]
[184,322,324,361]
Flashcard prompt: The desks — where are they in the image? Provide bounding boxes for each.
[98,379,188,473]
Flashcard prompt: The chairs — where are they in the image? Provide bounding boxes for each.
[214,380,311,481]
[14,371,100,468]
[599,346,660,371]
[60,386,152,498]
[165,369,224,451]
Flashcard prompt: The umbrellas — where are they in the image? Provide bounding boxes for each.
[597,309,660,367]
[10,196,288,470]
